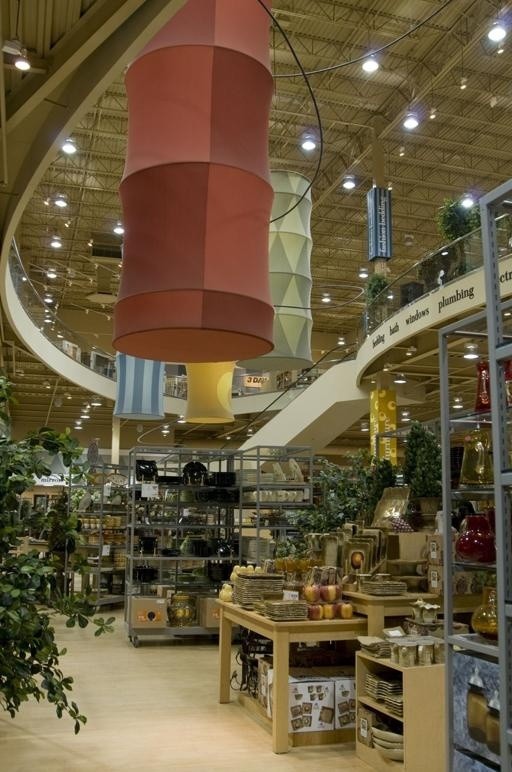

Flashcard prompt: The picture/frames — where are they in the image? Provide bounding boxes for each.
[34,493,49,511]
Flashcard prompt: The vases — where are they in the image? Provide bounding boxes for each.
[458,426,494,490]
[471,587,498,643]
[473,360,489,416]
[453,513,497,564]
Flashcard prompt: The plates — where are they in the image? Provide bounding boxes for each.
[265,600,308,620]
[363,580,407,595]
[366,672,403,716]
[373,726,404,762]
[234,572,284,609]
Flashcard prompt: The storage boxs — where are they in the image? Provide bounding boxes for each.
[200,597,238,628]
[313,665,356,730]
[127,595,167,628]
[266,666,334,731]
[157,584,175,597]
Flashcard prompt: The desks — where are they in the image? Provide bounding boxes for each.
[214,598,368,754]
[341,590,437,639]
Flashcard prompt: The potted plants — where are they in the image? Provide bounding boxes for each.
[408,442,443,521]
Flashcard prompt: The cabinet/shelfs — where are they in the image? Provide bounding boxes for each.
[67,461,135,616]
[438,177,510,770]
[123,443,315,647]
[477,180,510,683]
[355,649,446,772]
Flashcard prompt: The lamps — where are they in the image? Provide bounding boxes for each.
[62,139,77,155]
[55,194,67,207]
[47,267,58,279]
[301,133,316,152]
[44,293,53,304]
[113,209,125,235]
[404,112,419,131]
[343,168,355,190]
[362,56,379,74]
[460,191,474,208]
[3,39,31,71]
[50,234,62,248]
[488,22,507,42]
